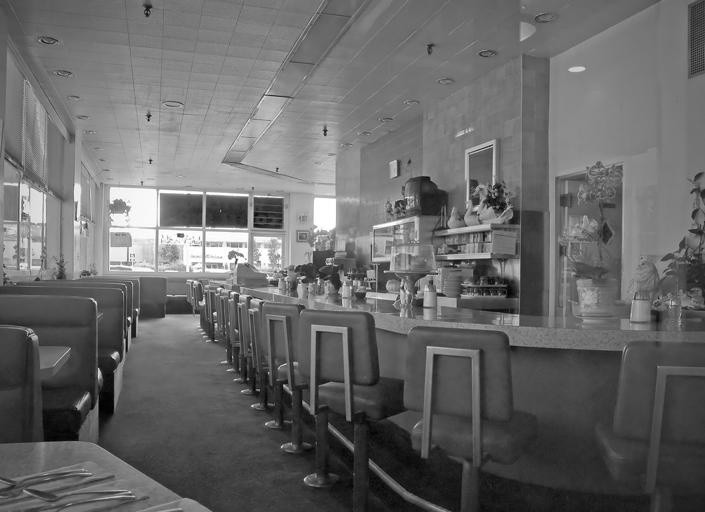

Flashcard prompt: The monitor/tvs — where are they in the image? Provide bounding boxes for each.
[404,175,449,216]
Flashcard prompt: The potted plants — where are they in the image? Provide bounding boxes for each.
[659,171,705,310]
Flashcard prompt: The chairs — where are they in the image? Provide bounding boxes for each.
[185,280,220,344]
[229,291,249,383]
[236,295,261,395]
[220,289,241,373]
[261,302,313,455]
[247,299,275,411]
[299,309,403,512]
[403,326,538,512]
[215,288,233,365]
[596,341,705,512]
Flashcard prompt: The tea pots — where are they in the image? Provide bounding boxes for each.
[385,278,404,293]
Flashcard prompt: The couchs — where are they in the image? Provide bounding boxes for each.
[0,279,140,444]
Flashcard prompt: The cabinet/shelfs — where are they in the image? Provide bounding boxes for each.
[372,216,447,273]
[431,224,518,310]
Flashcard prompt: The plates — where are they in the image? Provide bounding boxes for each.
[443,275,462,298]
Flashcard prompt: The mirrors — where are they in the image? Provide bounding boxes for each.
[463,140,499,212]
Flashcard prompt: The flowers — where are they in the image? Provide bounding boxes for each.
[569,161,623,280]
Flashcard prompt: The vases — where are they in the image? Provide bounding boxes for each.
[576,279,619,320]
